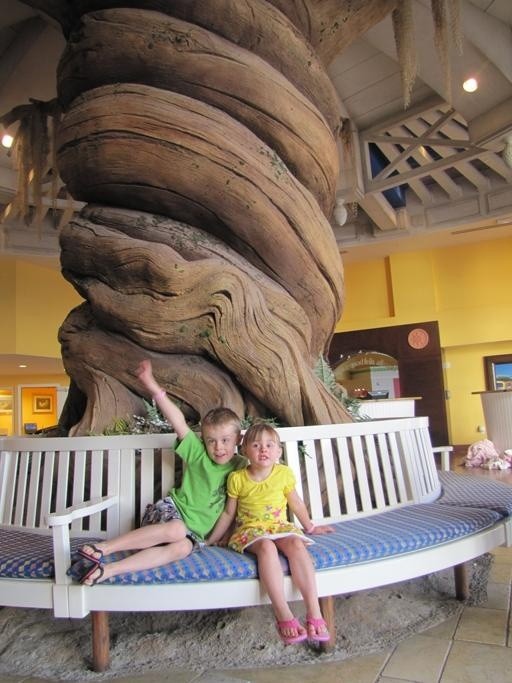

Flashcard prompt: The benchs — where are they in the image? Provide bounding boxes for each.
[0,417,511,671]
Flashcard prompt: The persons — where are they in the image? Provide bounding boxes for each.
[78,359,250,586]
[195,422,337,644]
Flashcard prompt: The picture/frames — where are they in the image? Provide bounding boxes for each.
[32,394,53,412]
[481,351,512,392]
[0,394,13,413]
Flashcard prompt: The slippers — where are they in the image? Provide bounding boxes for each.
[305,613,330,641]
[77,542,103,564]
[78,563,104,587]
[274,615,308,644]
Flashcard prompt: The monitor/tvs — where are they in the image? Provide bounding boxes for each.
[368,390,389,399]
